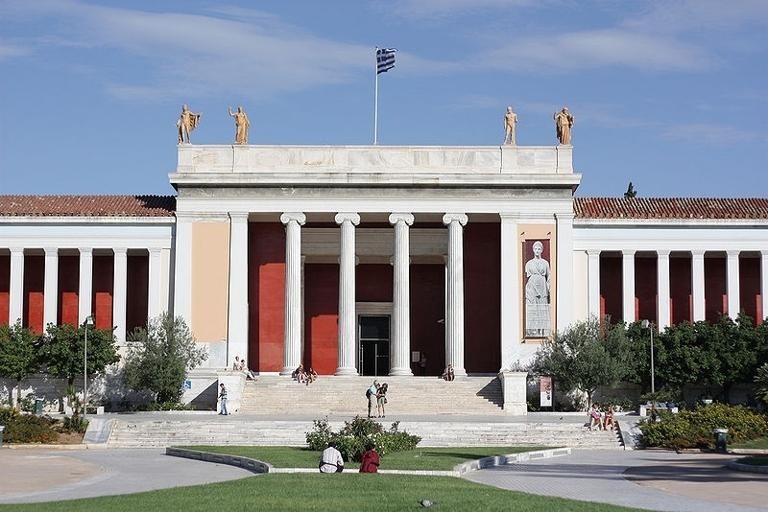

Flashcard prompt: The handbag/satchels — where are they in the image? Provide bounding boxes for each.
[337,464,343,472]
[384,398,387,403]
[592,412,599,420]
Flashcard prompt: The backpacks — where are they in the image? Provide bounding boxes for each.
[365,389,370,399]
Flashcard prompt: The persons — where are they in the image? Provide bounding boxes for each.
[376,383,388,418]
[603,404,615,431]
[586,403,603,432]
[358,444,379,474]
[318,442,344,474]
[502,105,518,145]
[232,356,257,381]
[443,364,454,381]
[227,105,250,145]
[552,107,574,145]
[524,241,550,334]
[293,365,318,386]
[175,103,202,144]
[217,383,228,415]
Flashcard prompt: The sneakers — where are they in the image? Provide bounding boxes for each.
[368,415,385,418]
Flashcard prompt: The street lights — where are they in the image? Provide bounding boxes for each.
[640,319,655,394]
[84,317,97,415]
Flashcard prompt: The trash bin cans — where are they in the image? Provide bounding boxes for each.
[714,429,728,453]
[35,400,43,414]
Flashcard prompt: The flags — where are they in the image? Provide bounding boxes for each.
[376,47,399,75]
